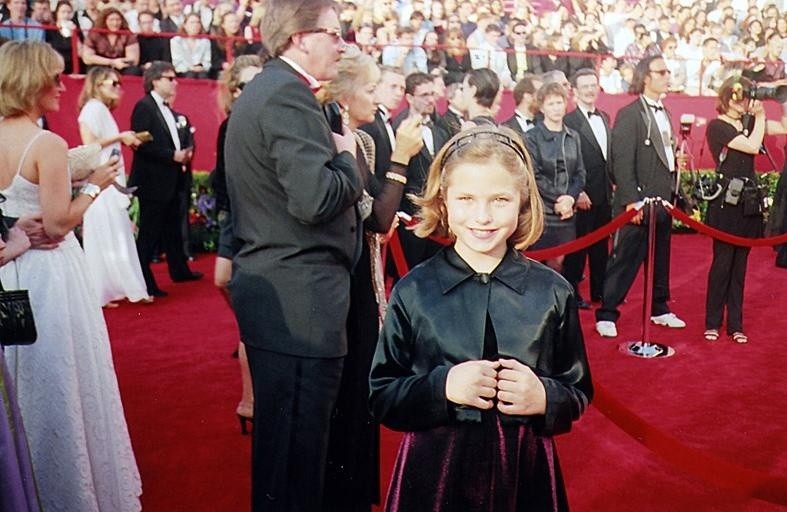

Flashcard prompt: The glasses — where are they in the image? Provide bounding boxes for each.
[160,76,177,81]
[649,69,672,75]
[103,80,123,87]
[292,25,342,37]
[233,83,253,91]
[52,73,60,86]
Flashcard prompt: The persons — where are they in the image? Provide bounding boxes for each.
[459,67,502,130]
[368,125,593,510]
[236,336,262,431]
[372,65,407,181]
[563,67,627,309]
[0,211,65,512]
[774,85,787,269]
[530,69,572,125]
[0,40,145,512]
[75,65,154,309]
[62,130,154,181]
[229,0,361,511]
[315,44,424,510]
[703,75,763,344]
[503,78,540,136]
[213,55,279,358]
[440,81,463,137]
[595,55,687,337]
[152,91,195,264]
[391,74,450,281]
[524,83,586,271]
[131,62,202,297]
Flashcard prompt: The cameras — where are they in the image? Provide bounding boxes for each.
[742,83,787,136]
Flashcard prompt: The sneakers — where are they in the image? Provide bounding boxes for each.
[594,319,620,337]
[651,312,687,328]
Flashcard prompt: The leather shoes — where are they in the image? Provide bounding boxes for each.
[148,289,167,297]
[575,300,592,312]
[173,271,206,282]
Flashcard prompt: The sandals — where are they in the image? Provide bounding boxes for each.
[702,328,721,342]
[731,331,748,345]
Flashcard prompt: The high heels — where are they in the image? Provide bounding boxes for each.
[236,401,253,434]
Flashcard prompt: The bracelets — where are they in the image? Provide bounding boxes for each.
[78,183,100,199]
[384,171,406,183]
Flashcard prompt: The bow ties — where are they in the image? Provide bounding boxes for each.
[423,120,433,129]
[587,108,595,119]
[525,118,537,126]
[162,101,170,107]
[650,105,664,112]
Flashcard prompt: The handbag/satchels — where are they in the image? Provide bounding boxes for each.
[0,260,38,347]
